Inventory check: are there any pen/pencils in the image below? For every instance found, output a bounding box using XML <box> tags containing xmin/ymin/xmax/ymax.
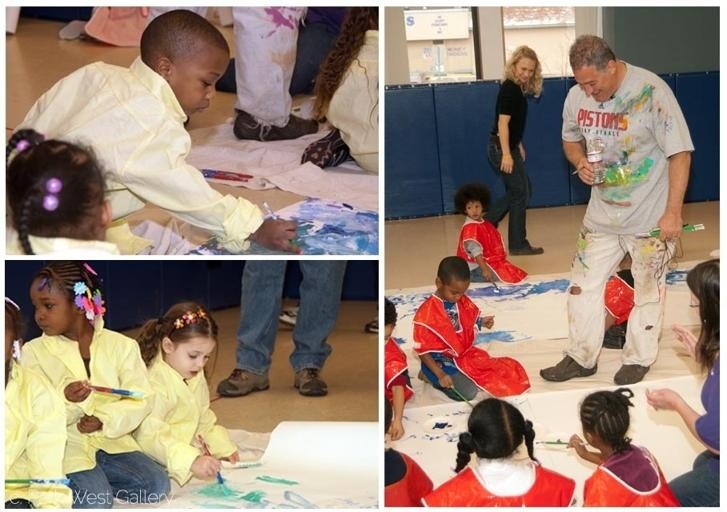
<box><xmin>262</xmin><ymin>201</ymin><xmax>296</xmax><ymax>245</ymax></box>
<box><xmin>569</xmin><ymin>166</ymin><xmax>584</xmax><ymax>176</ymax></box>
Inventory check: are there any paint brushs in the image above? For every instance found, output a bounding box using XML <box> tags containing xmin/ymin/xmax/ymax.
<box><xmin>493</xmin><ymin>282</ymin><xmax>499</xmax><ymax>291</ymax></box>
<box><xmin>535</xmin><ymin>441</ymin><xmax>591</xmax><ymax>445</ymax></box>
<box><xmin>449</xmin><ymin>385</ymin><xmax>475</xmax><ymax>410</ymax></box>
<box><xmin>89</xmin><ymin>386</ymin><xmax>144</xmax><ymax>397</ymax></box>
<box><xmin>5</xmin><ymin>479</ymin><xmax>75</xmax><ymax>488</ymax></box>
<box><xmin>199</xmin><ymin>434</ymin><xmax>231</xmax><ymax>495</ymax></box>
<box><xmin>634</xmin><ymin>223</ymin><xmax>705</xmax><ymax>238</ymax></box>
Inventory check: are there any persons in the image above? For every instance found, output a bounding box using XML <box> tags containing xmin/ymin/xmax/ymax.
<box><xmin>411</xmin><ymin>253</ymin><xmax>496</xmax><ymax>402</ymax></box>
<box><xmin>644</xmin><ymin>256</ymin><xmax>718</xmax><ymax>505</ymax></box>
<box><xmin>5</xmin><ymin>294</ymin><xmax>74</xmax><ymax>509</ymax></box>
<box><xmin>57</xmin><ymin>9</ymin><xmax>379</xmax><ymax>179</ymax></box>
<box><xmin>418</xmin><ymin>396</ymin><xmax>582</xmax><ymax>507</ymax></box>
<box><xmin>7</xmin><ymin>126</ymin><xmax>126</xmax><ymax>256</ymax></box>
<box><xmin>19</xmin><ymin>259</ymin><xmax>170</xmax><ymax>510</ymax></box>
<box><xmin>213</xmin><ymin>259</ymin><xmax>350</xmax><ymax>397</ymax></box>
<box><xmin>276</xmin><ymin>273</ymin><xmax>378</xmax><ymax>334</ymax></box>
<box><xmin>7</xmin><ymin>9</ymin><xmax>308</xmax><ymax>256</ymax></box>
<box><xmin>383</xmin><ymin>397</ymin><xmax>433</xmax><ymax>506</ymax></box>
<box><xmin>451</xmin><ymin>182</ymin><xmax>529</xmax><ymax>286</ymax></box>
<box><xmin>564</xmin><ymin>385</ymin><xmax>684</xmax><ymax>506</ymax></box>
<box><xmin>481</xmin><ymin>45</ymin><xmax>545</xmax><ymax>256</ymax></box>
<box><xmin>603</xmin><ymin>250</ymin><xmax>635</xmax><ymax>350</ymax></box>
<box><xmin>383</xmin><ymin>295</ymin><xmax>414</xmax><ymax>441</ymax></box>
<box><xmin>537</xmin><ymin>33</ymin><xmax>694</xmax><ymax>386</ymax></box>
<box><xmin>128</xmin><ymin>298</ymin><xmax>241</xmax><ymax>487</ymax></box>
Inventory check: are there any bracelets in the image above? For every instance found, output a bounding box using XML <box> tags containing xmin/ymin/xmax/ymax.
<box><xmin>502</xmin><ymin>155</ymin><xmax>512</xmax><ymax>160</ymax></box>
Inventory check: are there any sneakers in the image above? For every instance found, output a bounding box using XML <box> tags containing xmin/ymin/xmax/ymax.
<box><xmin>615</xmin><ymin>360</ymin><xmax>648</xmax><ymax>385</ymax></box>
<box><xmin>294</xmin><ymin>367</ymin><xmax>329</xmax><ymax>397</ymax></box>
<box><xmin>278</xmin><ymin>306</ymin><xmax>300</xmax><ymax>326</ymax></box>
<box><xmin>365</xmin><ymin>320</ymin><xmax>379</xmax><ymax>333</ymax></box>
<box><xmin>230</xmin><ymin>111</ymin><xmax>319</xmax><ymax>141</ymax></box>
<box><xmin>418</xmin><ymin>370</ymin><xmax>432</xmax><ymax>384</ymax></box>
<box><xmin>508</xmin><ymin>245</ymin><xmax>543</xmax><ymax>255</ymax></box>
<box><xmin>541</xmin><ymin>356</ymin><xmax>597</xmax><ymax>380</ymax></box>
<box><xmin>301</xmin><ymin>126</ymin><xmax>352</xmax><ymax>170</ymax></box>
<box><xmin>217</xmin><ymin>369</ymin><xmax>269</xmax><ymax>398</ymax></box>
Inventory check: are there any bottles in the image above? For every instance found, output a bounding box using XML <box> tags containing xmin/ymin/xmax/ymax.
<box><xmin>585</xmin><ymin>137</ymin><xmax>606</xmax><ymax>185</ymax></box>
